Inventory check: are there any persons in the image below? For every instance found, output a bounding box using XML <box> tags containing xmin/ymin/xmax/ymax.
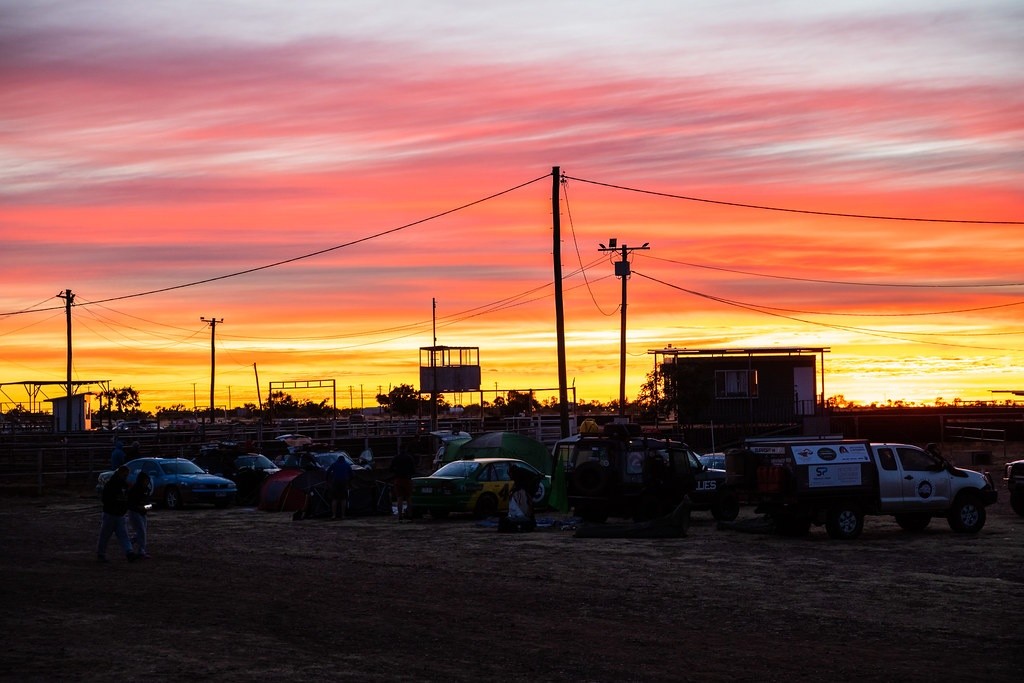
<box><xmin>207</xmin><ymin>439</ymin><xmax>354</xmax><ymax>521</ymax></box>
<box><xmin>431</xmin><ymin>441</ymin><xmax>447</xmax><ymax>471</ymax></box>
<box><xmin>926</xmin><ymin>443</ymin><xmax>945</xmax><ymax>459</ymax></box>
<box><xmin>390</xmin><ymin>444</ymin><xmax>415</xmax><ymax>524</ymax></box>
<box><xmin>95</xmin><ymin>442</ymin><xmax>153</xmax><ymax>561</ymax></box>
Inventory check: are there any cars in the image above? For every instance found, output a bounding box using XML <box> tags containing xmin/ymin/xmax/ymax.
<box><xmin>94</xmin><ymin>457</ymin><xmax>238</xmax><ymax>509</ymax></box>
<box><xmin>95</xmin><ymin>419</ymin><xmax>165</xmax><ymax>433</ymax></box>
<box><xmin>410</xmin><ymin>456</ymin><xmax>553</xmax><ymax>520</ymax></box>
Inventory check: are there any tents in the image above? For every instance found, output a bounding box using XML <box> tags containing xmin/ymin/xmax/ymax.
<box><xmin>455</xmin><ymin>431</ymin><xmax>567</xmax><ymax>512</ymax></box>
<box><xmin>260</xmin><ymin>467</ymin><xmax>390</xmax><ymax>518</ymax></box>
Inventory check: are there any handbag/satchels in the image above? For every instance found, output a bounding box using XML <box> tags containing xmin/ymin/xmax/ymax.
<box><xmin>499</xmin><ymin>515</ymin><xmax>531</xmax><ymax>532</ymax></box>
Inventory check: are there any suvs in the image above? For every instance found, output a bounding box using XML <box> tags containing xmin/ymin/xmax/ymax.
<box><xmin>566</xmin><ymin>419</ymin><xmax>741</xmax><ymax>525</ymax></box>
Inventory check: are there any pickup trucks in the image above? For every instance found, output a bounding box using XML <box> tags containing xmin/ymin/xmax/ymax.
<box><xmin>720</xmin><ymin>434</ymin><xmax>997</xmax><ymax>541</ymax></box>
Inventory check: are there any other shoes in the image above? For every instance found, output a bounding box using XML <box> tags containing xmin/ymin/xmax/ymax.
<box><xmin>128</xmin><ymin>552</ymin><xmax>144</xmax><ymax>561</ymax></box>
<box><xmin>144</xmin><ymin>554</ymin><xmax>152</xmax><ymax>559</ymax></box>
<box><xmin>96</xmin><ymin>555</ymin><xmax>108</xmax><ymax>562</ymax></box>
